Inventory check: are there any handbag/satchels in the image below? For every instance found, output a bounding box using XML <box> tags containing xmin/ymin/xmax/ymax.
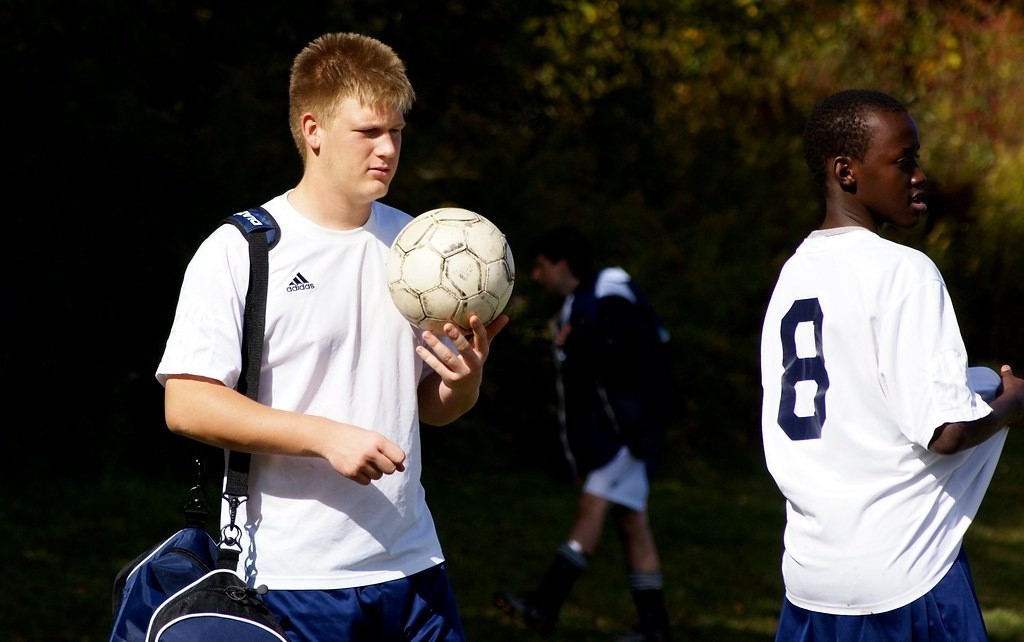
<box><xmin>106</xmin><ymin>524</ymin><xmax>291</xmax><ymax>642</ymax></box>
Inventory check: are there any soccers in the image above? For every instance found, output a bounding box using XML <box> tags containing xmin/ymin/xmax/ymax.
<box><xmin>383</xmin><ymin>205</ymin><xmax>520</xmax><ymax>339</ymax></box>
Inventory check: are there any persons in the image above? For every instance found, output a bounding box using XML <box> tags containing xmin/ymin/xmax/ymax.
<box><xmin>491</xmin><ymin>240</ymin><xmax>683</xmax><ymax>642</ymax></box>
<box><xmin>760</xmin><ymin>89</ymin><xmax>1024</xmax><ymax>642</ymax></box>
<box><xmin>156</xmin><ymin>32</ymin><xmax>509</xmax><ymax>642</ymax></box>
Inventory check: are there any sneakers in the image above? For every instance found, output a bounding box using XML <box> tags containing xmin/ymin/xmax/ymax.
<box><xmin>491</xmin><ymin>590</ymin><xmax>562</xmax><ymax>639</ymax></box>
<box><xmin>616</xmin><ymin>633</ymin><xmax>674</xmax><ymax>642</ymax></box>
